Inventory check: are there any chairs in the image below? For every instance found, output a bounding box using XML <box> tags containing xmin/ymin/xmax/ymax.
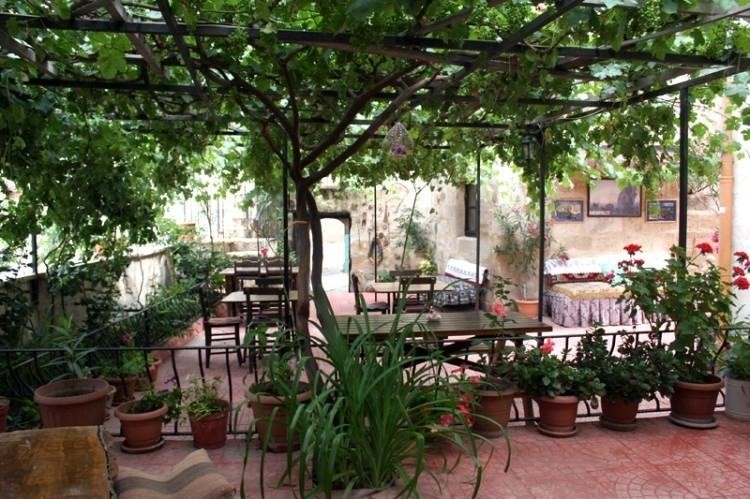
<box><xmin>201</xmin><ymin>256</ymin><xmax>437</xmax><ymax>371</ymax></box>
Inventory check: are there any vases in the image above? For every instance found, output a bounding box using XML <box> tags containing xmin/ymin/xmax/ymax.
<box><xmin>534</xmin><ymin>394</ymin><xmax>581</xmax><ymax>437</ymax></box>
<box><xmin>668</xmin><ymin>374</ymin><xmax>726</xmax><ymax>429</ymax></box>
<box><xmin>463</xmin><ymin>377</ymin><xmax>517</xmax><ymax>436</ymax></box>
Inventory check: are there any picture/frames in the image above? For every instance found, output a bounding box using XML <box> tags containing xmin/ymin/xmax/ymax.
<box><xmin>586</xmin><ymin>176</ymin><xmax>641</xmax><ymax>218</ymax></box>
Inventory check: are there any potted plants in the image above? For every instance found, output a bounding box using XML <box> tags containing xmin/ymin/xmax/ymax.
<box><xmin>35</xmin><ymin>318</ymin><xmax>312</xmax><ymax>453</ymax></box>
<box><xmin>586</xmin><ymin>326</ymin><xmax>660</xmax><ymax>425</ymax></box>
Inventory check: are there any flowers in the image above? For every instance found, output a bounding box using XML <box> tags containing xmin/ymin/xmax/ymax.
<box><xmin>527</xmin><ymin>340</ymin><xmax>587</xmax><ymax>398</ymax></box>
<box><xmin>613</xmin><ymin>242</ymin><xmax>750</xmax><ymax>377</ymax></box>
<box><xmin>437</xmin><ymin>301</ymin><xmax>522</xmax><ymax>427</ymax></box>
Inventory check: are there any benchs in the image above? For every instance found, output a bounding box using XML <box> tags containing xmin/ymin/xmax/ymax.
<box><xmin>434</xmin><ymin>257</ymin><xmax>488</xmax><ymax>306</ymax></box>
